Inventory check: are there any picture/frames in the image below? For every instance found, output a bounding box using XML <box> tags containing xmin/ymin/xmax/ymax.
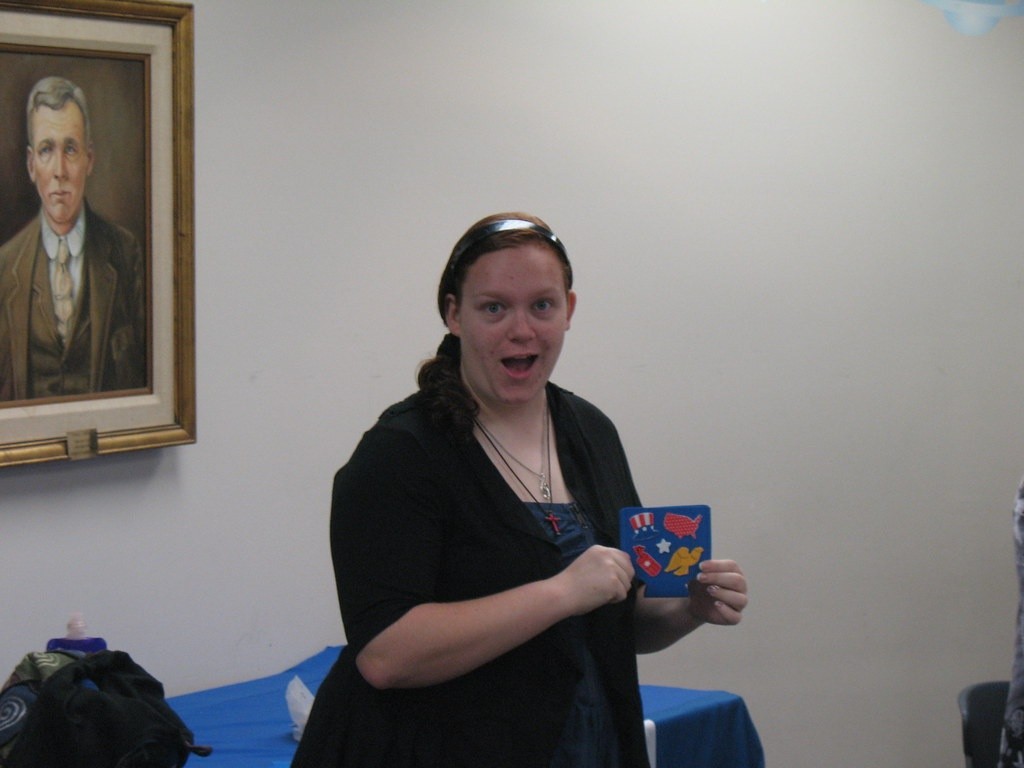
<box><xmin>0</xmin><ymin>0</ymin><xmax>197</xmax><ymax>466</ymax></box>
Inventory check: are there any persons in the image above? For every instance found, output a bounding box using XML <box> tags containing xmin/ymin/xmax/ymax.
<box><xmin>1005</xmin><ymin>479</ymin><xmax>1024</xmax><ymax>750</ymax></box>
<box><xmin>288</xmin><ymin>214</ymin><xmax>748</xmax><ymax>768</ymax></box>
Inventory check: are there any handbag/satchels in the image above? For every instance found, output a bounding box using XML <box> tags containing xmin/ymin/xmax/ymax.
<box><xmin>8</xmin><ymin>651</ymin><xmax>212</xmax><ymax>768</ymax></box>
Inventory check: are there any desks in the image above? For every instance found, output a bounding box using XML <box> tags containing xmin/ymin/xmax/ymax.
<box><xmin>164</xmin><ymin>644</ymin><xmax>765</xmax><ymax>768</ymax></box>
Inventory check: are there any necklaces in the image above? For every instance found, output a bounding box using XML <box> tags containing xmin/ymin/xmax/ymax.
<box><xmin>474</xmin><ymin>384</ymin><xmax>561</xmax><ymax>537</ymax></box>
